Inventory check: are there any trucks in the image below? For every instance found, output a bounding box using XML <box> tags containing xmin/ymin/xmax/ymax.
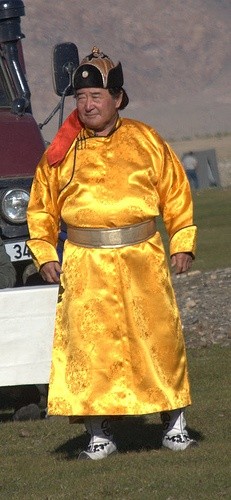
<box><xmin>0</xmin><ymin>0</ymin><xmax>79</xmax><ymax>412</ymax></box>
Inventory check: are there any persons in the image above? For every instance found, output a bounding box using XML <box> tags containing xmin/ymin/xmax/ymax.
<box><xmin>25</xmin><ymin>46</ymin><xmax>198</xmax><ymax>460</ymax></box>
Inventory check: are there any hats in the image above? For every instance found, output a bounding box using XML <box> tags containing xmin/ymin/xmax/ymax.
<box><xmin>71</xmin><ymin>46</ymin><xmax>123</xmax><ymax>90</ymax></box>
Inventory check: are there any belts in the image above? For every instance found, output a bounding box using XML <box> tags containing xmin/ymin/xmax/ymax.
<box><xmin>66</xmin><ymin>219</ymin><xmax>155</xmax><ymax>249</ymax></box>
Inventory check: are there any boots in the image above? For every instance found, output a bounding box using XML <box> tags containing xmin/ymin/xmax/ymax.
<box><xmin>160</xmin><ymin>406</ymin><xmax>200</xmax><ymax>451</ymax></box>
<box><xmin>77</xmin><ymin>413</ymin><xmax>117</xmax><ymax>460</ymax></box>
